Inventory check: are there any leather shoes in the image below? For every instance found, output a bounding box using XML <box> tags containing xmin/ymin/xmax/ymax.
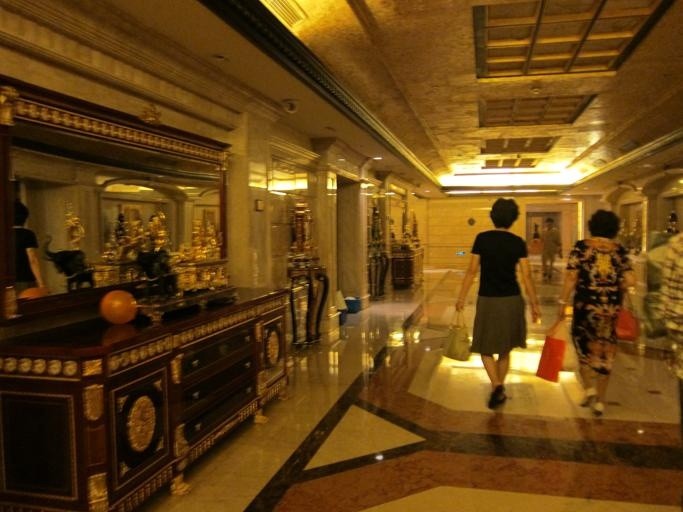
<box><xmin>489</xmin><ymin>383</ymin><xmax>508</xmax><ymax>410</ymax></box>
<box><xmin>578</xmin><ymin>385</ymin><xmax>608</xmax><ymax>418</ymax></box>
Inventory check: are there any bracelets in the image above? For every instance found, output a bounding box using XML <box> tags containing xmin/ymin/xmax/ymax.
<box><xmin>557</xmin><ymin>299</ymin><xmax>567</xmax><ymax>305</ymax></box>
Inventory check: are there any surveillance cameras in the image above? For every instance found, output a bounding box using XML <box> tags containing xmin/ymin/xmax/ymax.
<box><xmin>282</xmin><ymin>99</ymin><xmax>300</xmax><ymax>114</ymax></box>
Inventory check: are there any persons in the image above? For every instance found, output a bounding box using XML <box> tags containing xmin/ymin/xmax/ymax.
<box><xmin>534</xmin><ymin>218</ymin><xmax>563</xmax><ymax>278</ymax></box>
<box><xmin>556</xmin><ymin>210</ymin><xmax>637</xmax><ymax>415</ymax></box>
<box><xmin>13</xmin><ymin>201</ymin><xmax>46</xmax><ymax>288</ymax></box>
<box><xmin>454</xmin><ymin>196</ymin><xmax>540</xmax><ymax>411</ymax></box>
<box><xmin>654</xmin><ymin>227</ymin><xmax>683</xmax><ymax>447</ymax></box>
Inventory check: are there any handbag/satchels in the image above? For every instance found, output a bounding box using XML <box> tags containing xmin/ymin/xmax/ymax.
<box><xmin>615</xmin><ymin>289</ymin><xmax>642</xmax><ymax>342</ymax></box>
<box><xmin>442</xmin><ymin>309</ymin><xmax>472</xmax><ymax>361</ymax></box>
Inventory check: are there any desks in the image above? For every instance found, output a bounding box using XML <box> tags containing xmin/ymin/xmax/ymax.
<box><xmin>285</xmin><ymin>264</ymin><xmax>330</xmax><ymax>346</ymax></box>
<box><xmin>367</xmin><ymin>250</ymin><xmax>389</xmax><ymax>301</ymax></box>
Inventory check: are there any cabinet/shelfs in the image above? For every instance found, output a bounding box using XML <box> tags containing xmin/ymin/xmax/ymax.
<box><xmin>0</xmin><ymin>287</ymin><xmax>294</xmax><ymax>511</ymax></box>
<box><xmin>392</xmin><ymin>247</ymin><xmax>426</xmax><ymax>289</ymax></box>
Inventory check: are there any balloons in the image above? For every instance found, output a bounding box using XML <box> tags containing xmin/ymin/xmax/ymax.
<box><xmin>18</xmin><ymin>286</ymin><xmax>45</xmax><ymax>299</ymax></box>
<box><xmin>98</xmin><ymin>290</ymin><xmax>139</xmax><ymax>325</ymax></box>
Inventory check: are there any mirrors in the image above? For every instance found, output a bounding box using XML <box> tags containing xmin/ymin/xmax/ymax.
<box><xmin>0</xmin><ymin>73</ymin><xmax>231</xmax><ymax>324</ymax></box>
